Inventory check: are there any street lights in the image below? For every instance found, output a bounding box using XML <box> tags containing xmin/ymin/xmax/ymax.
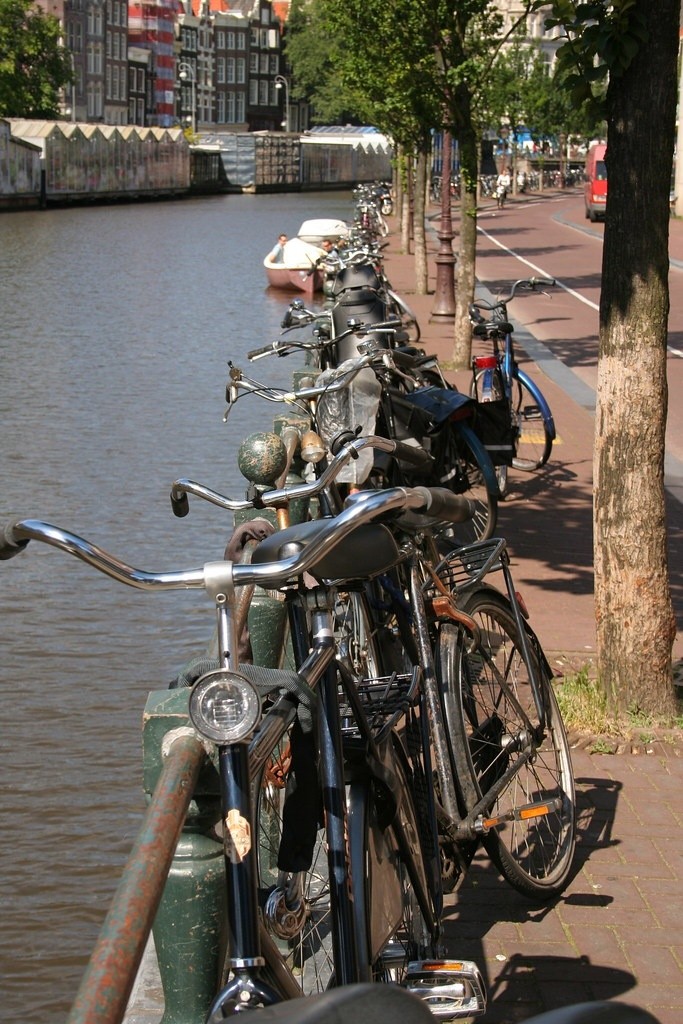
<box><xmin>274</xmin><ymin>75</ymin><xmax>291</xmax><ymax>133</ymax></box>
<box><xmin>178</xmin><ymin>62</ymin><xmax>199</xmax><ymax>136</ymax></box>
<box><xmin>52</xmin><ymin>44</ymin><xmax>77</xmax><ymax>126</ymax></box>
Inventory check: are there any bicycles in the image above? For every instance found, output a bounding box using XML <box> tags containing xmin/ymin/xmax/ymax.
<box><xmin>0</xmin><ymin>164</ymin><xmax>588</xmax><ymax>1024</ymax></box>
<box><xmin>493</xmin><ymin>181</ymin><xmax>507</xmax><ymax>214</ymax></box>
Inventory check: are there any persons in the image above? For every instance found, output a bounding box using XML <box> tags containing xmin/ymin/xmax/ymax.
<box><xmin>496</xmin><ymin>170</ymin><xmax>510</xmax><ymax>210</ymax></box>
<box><xmin>270</xmin><ymin>234</ymin><xmax>287</xmax><ymax>263</ymax></box>
<box><xmin>322</xmin><ymin>239</ymin><xmax>348</xmax><ymax>272</ymax></box>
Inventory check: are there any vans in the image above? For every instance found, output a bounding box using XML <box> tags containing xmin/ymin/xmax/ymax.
<box><xmin>581</xmin><ymin>143</ymin><xmax>609</xmax><ymax>222</ymax></box>
